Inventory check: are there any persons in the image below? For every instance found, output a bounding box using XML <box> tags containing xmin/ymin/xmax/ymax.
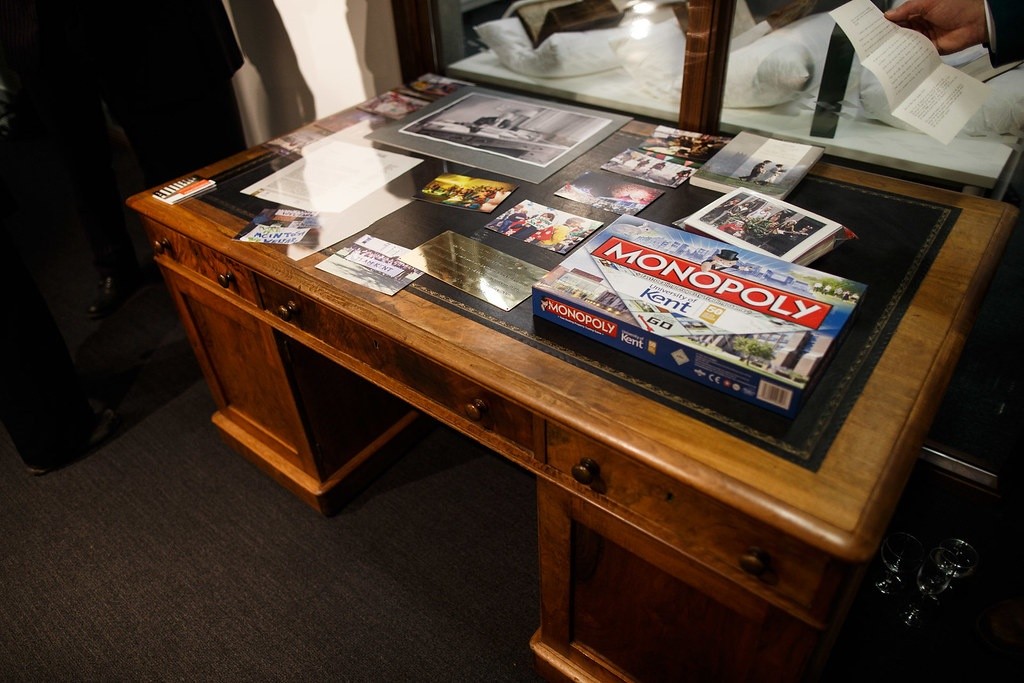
<box><xmin>608</xmin><ymin>151</ymin><xmax>692</xmax><ymax>186</ymax></box>
<box><xmin>701</xmin><ymin>198</ymin><xmax>813</xmax><ymax>242</ymax></box>
<box><xmin>884</xmin><ymin>0</ymin><xmax>1024</xmax><ymax>69</ymax></box>
<box><xmin>342</xmin><ymin>245</ymin><xmax>415</xmax><ymax>282</ymax></box>
<box><xmin>424</xmin><ymin>179</ymin><xmax>497</xmax><ymax>211</ymax></box>
<box><xmin>740</xmin><ymin>159</ymin><xmax>788</xmax><ymax>186</ymax></box>
<box><xmin>642</xmin><ymin>132</ymin><xmax>730</xmax><ymax>160</ymax></box>
<box><xmin>470</xmin><ymin>112</ymin><xmax>514</xmax><ymax>133</ymax></box>
<box><xmin>489</xmin><ymin>204</ymin><xmax>596</xmax><ymax>252</ymax></box>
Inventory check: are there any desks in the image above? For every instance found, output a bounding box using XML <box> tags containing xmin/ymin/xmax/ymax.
<box><xmin>391</xmin><ymin>0</ymin><xmax>1024</xmax><ymax>202</ymax></box>
<box><xmin>125</xmin><ymin>70</ymin><xmax>1021</xmax><ymax>683</ymax></box>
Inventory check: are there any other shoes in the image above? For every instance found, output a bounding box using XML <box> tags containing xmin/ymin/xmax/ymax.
<box><xmin>27</xmin><ymin>408</ymin><xmax>119</xmax><ymax>475</ymax></box>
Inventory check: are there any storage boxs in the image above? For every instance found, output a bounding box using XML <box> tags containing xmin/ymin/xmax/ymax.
<box><xmin>532</xmin><ymin>212</ymin><xmax>869</xmax><ymax>420</ymax></box>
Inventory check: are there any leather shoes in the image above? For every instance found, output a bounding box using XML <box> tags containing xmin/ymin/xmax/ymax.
<box><xmin>88</xmin><ymin>275</ymin><xmax>132</xmax><ymax>317</ymax></box>
